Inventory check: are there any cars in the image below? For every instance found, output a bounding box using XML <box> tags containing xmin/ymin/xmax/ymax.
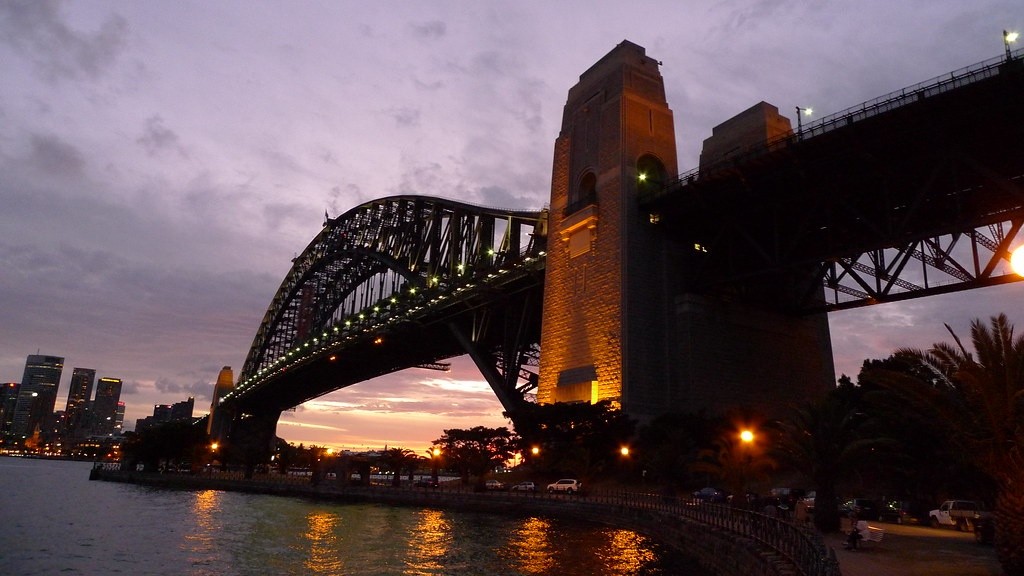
<box><xmin>512</xmin><ymin>481</ymin><xmax>535</xmax><ymax>492</ymax></box>
<box><xmin>751</xmin><ymin>493</ymin><xmax>798</xmax><ymax>513</ymax></box>
<box><xmin>801</xmin><ymin>491</ymin><xmax>817</xmax><ymax>513</ymax></box>
<box><xmin>876</xmin><ymin>505</ymin><xmax>924</xmax><ymax>525</ymax></box>
<box><xmin>485</xmin><ymin>479</ymin><xmax>504</xmax><ymax>491</ymax></box>
<box><xmin>929</xmin><ymin>499</ymin><xmax>984</xmax><ymax>533</ymax></box>
<box><xmin>693</xmin><ymin>486</ymin><xmax>726</xmax><ymax>503</ymax></box>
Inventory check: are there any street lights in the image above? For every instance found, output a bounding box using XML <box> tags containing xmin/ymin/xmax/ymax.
<box><xmin>1002</xmin><ymin>29</ymin><xmax>1017</xmax><ymax>62</ymax></box>
<box><xmin>796</xmin><ymin>105</ymin><xmax>812</xmax><ymax>143</ymax></box>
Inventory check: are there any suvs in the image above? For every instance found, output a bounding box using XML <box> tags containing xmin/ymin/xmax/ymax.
<box><xmin>547</xmin><ymin>479</ymin><xmax>583</xmax><ymax>495</ymax></box>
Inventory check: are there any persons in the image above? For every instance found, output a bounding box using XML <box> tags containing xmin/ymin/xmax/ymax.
<box><xmin>847</xmin><ymin>503</ymin><xmax>859</xmax><ymax>529</ymax></box>
<box><xmin>792</xmin><ymin>496</ymin><xmax>809</xmax><ymax>527</ymax></box>
<box><xmin>744</xmin><ymin>487</ymin><xmax>757</xmax><ymax>511</ymax></box>
<box><xmin>576</xmin><ymin>480</ymin><xmax>583</xmax><ymax>496</ymax></box>
<box><xmin>763</xmin><ymin>491</ymin><xmax>779</xmax><ymax>517</ymax></box>
